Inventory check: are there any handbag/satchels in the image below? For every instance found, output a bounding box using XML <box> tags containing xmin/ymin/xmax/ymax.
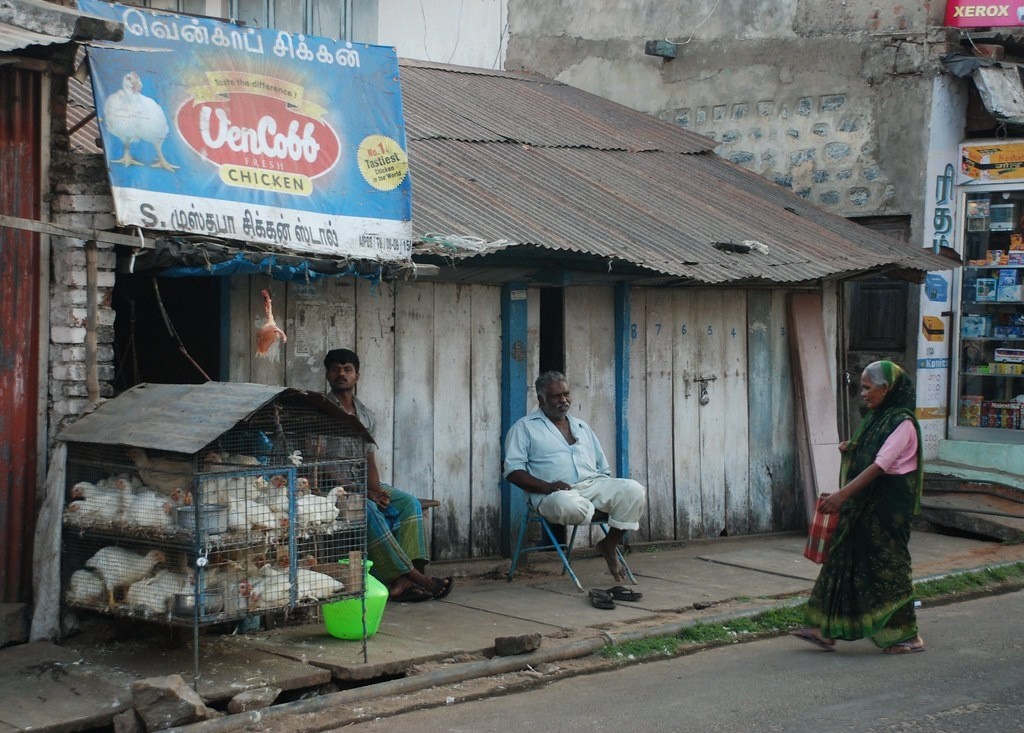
<box><xmin>804</xmin><ymin>492</ymin><xmax>839</xmax><ymax>564</ymax></box>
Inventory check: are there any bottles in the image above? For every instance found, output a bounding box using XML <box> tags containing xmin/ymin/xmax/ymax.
<box><xmin>321</xmin><ymin>559</ymin><xmax>389</xmax><ymax>640</ymax></box>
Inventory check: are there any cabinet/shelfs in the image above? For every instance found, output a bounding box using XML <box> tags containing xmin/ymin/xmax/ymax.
<box><xmin>948</xmin><ymin>139</ymin><xmax>1024</xmax><ymax>445</ymax></box>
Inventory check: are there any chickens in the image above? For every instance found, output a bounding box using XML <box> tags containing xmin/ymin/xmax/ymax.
<box><xmin>58</xmin><ymin>448</ymin><xmax>351</xmax><ymax>618</ymax></box>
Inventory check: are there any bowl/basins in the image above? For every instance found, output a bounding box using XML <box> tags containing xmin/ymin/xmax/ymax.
<box><xmin>175</xmin><ymin>503</ymin><xmax>228</xmax><ymax>534</ymax></box>
<box><xmin>174</xmin><ymin>592</ymin><xmax>224</xmax><ymax>618</ymax></box>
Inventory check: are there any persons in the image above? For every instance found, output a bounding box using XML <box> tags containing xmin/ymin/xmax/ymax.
<box><xmin>502</xmin><ymin>369</ymin><xmax>647</xmax><ymax>582</ymax></box>
<box><xmin>785</xmin><ymin>358</ymin><xmax>927</xmax><ymax>655</ymax></box>
<box><xmin>309</xmin><ymin>348</ymin><xmax>455</xmax><ymax>603</ymax></box>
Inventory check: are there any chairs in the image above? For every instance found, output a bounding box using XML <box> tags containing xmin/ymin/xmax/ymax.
<box><xmin>508</xmin><ymin>486</ymin><xmax>638</xmax><ymax>595</ymax></box>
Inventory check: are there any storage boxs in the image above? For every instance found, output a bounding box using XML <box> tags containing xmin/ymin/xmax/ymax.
<box><xmin>998</xmin><ymin>269</ymin><xmax>1017</xmax><ymax>286</ymax></box>
<box><xmin>997</xmin><ymin>286</ymin><xmax>1023</xmax><ymax>302</ymax></box>
<box><xmin>966</xmin><ymin>199</ymin><xmax>990</xmax><ymax>217</ymax></box>
<box><xmin>981</xmin><ymin>401</ymin><xmax>1024</xmax><ymax>430</ymax></box>
<box><xmin>960</xmin><ymin>317</ymin><xmax>991</xmax><ymax>337</ymax></box>
<box><xmin>976</xmin><ymin>278</ymin><xmax>997</xmax><ymax>302</ymax></box>
<box><xmin>958</xmin><ymin>396</ymin><xmax>984</xmax><ymax>427</ymax></box>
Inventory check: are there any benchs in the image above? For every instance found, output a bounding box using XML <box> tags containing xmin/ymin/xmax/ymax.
<box><xmin>418</xmin><ymin>496</ymin><xmax>442</xmax><ymax>570</ymax></box>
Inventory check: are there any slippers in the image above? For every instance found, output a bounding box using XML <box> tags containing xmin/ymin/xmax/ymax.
<box><xmin>431</xmin><ymin>576</ymin><xmax>455</xmax><ymax>598</ymax></box>
<box><xmin>792</xmin><ymin>628</ymin><xmax>836</xmax><ymax>651</ymax></box>
<box><xmin>883</xmin><ymin>642</ymin><xmax>926</xmax><ymax>654</ymax></box>
<box><xmin>390</xmin><ymin>584</ymin><xmax>433</xmax><ymax>603</ymax></box>
<box><xmin>588</xmin><ymin>589</ymin><xmax>616</xmax><ymax>608</ymax></box>
<box><xmin>607</xmin><ymin>585</ymin><xmax>642</xmax><ymax>600</ymax></box>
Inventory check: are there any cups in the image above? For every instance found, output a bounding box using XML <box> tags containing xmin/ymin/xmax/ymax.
<box><xmin>962</xmin><ymin>148</ymin><xmax>971</xmax><ymax>174</ymax></box>
<box><xmin>981</xmin><ymin>154</ymin><xmax>991</xmax><ymax>179</ymax></box>
